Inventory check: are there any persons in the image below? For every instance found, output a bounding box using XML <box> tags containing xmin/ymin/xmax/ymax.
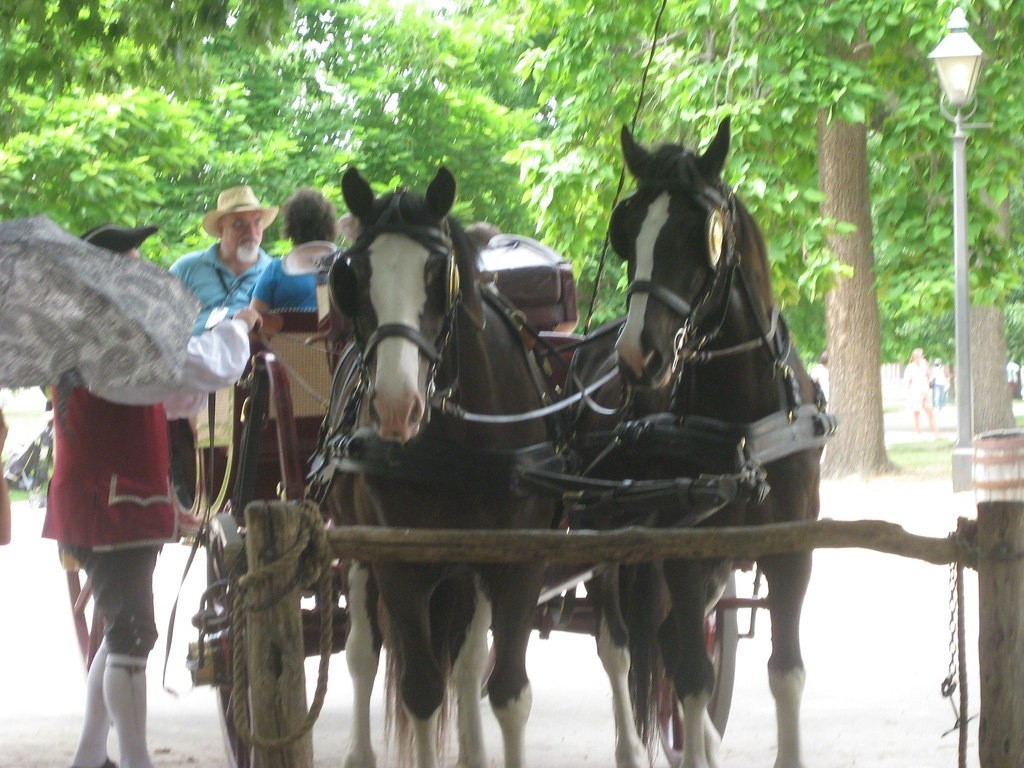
<box><xmin>251</xmin><ymin>185</ymin><xmax>344</xmax><ymax>306</ymax></box>
<box><xmin>931</xmin><ymin>355</ymin><xmax>1024</xmax><ymax>406</ymax></box>
<box><xmin>36</xmin><ymin>220</ymin><xmax>262</xmax><ymax>768</ymax></box>
<box><xmin>164</xmin><ymin>185</ymin><xmax>281</xmax><ymax>508</ymax></box>
<box><xmin>809</xmin><ymin>351</ymin><xmax>830</xmax><ymax>408</ymax></box>
<box><xmin>464</xmin><ymin>221</ymin><xmax>502</xmax><ymax>248</ymax></box>
<box><xmin>903</xmin><ymin>348</ymin><xmax>938</xmax><ymax>431</ymax></box>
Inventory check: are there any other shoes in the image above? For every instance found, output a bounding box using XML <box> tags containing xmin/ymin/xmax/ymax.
<box><xmin>103</xmin><ymin>758</ymin><xmax>120</xmax><ymax>768</ymax></box>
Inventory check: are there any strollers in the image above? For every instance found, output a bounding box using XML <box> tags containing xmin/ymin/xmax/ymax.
<box><xmin>3</xmin><ymin>418</ymin><xmax>54</xmax><ymax>509</ymax></box>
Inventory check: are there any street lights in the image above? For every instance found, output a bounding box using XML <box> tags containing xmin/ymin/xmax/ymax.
<box><xmin>928</xmin><ymin>5</ymin><xmax>985</xmax><ymax>518</ymax></box>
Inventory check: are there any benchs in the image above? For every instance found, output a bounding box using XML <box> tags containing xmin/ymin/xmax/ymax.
<box><xmin>215</xmin><ymin>265</ymin><xmax>580</xmax><ymax>518</ymax></box>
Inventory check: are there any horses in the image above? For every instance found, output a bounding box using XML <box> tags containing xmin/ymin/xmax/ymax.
<box><xmin>322</xmin><ymin>162</ymin><xmax>556</xmax><ymax>768</ymax></box>
<box><xmin>562</xmin><ymin>112</ymin><xmax>828</xmax><ymax>768</ymax></box>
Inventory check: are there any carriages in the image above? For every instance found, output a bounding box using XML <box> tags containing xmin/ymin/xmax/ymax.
<box><xmin>174</xmin><ymin>113</ymin><xmax>838</xmax><ymax>768</ymax></box>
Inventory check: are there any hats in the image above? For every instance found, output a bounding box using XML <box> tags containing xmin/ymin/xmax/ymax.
<box><xmin>81</xmin><ymin>226</ymin><xmax>157</xmax><ymax>252</ymax></box>
<box><xmin>202</xmin><ymin>185</ymin><xmax>280</xmax><ymax>239</ymax></box>
